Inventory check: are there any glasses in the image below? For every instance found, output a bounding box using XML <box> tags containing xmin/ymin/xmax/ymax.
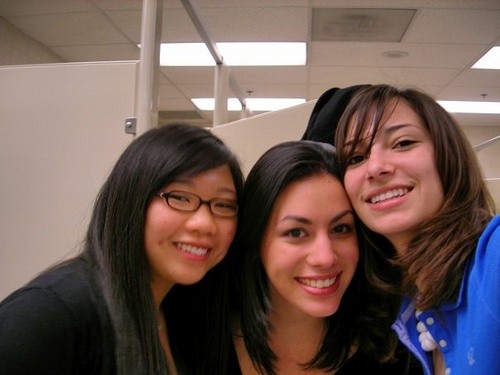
<box><xmin>156</xmin><ymin>190</ymin><xmax>239</xmax><ymax>218</ymax></box>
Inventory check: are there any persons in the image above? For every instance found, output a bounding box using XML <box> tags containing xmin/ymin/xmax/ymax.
<box><xmin>227</xmin><ymin>140</ymin><xmax>370</xmax><ymax>375</ymax></box>
<box><xmin>336</xmin><ymin>84</ymin><xmax>500</xmax><ymax>375</ymax></box>
<box><xmin>0</xmin><ymin>124</ymin><xmax>245</xmax><ymax>375</ymax></box>
